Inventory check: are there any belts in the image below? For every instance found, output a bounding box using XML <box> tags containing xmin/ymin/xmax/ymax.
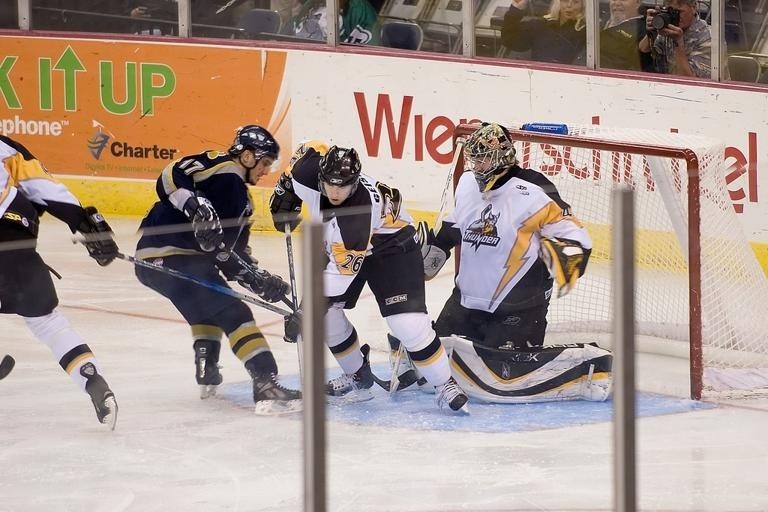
<box><xmin>249</xmin><ymin>268</ymin><xmax>293</xmax><ymax>303</ymax></box>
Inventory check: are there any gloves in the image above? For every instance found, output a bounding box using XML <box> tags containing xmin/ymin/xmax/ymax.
<box><xmin>68</xmin><ymin>202</ymin><xmax>119</xmax><ymax>267</ymax></box>
<box><xmin>180</xmin><ymin>194</ymin><xmax>224</xmax><ymax>269</ymax></box>
<box><xmin>271</xmin><ymin>171</ymin><xmax>306</xmax><ymax>232</ymax></box>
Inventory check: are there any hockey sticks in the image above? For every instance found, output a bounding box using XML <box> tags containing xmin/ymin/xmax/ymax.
<box><xmin>387</xmin><ymin>136</ymin><xmax>466</xmax><ymax>398</ymax></box>
<box><xmin>220</xmin><ymin>242</ymin><xmax>418</xmax><ymax>392</ymax></box>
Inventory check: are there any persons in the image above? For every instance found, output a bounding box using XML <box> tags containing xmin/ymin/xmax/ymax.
<box><xmin>0</xmin><ymin>134</ymin><xmax>119</xmax><ymax>433</ymax></box>
<box><xmin>420</xmin><ymin>120</ymin><xmax>614</xmax><ymax>403</ymax></box>
<box><xmin>135</xmin><ymin>124</ymin><xmax>303</xmax><ymax>416</ymax></box>
<box><xmin>66</xmin><ymin>0</ymin><xmax>732</xmax><ymax>81</ymax></box>
<box><xmin>268</xmin><ymin>138</ymin><xmax>470</xmax><ymax>416</ymax></box>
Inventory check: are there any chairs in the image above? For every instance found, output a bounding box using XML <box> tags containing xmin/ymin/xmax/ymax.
<box><xmin>17</xmin><ymin>1</ymin><xmax>767</xmax><ymax>89</ymax></box>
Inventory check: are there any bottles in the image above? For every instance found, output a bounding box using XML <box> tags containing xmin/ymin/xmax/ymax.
<box><xmin>518</xmin><ymin>122</ymin><xmax>568</xmax><ymax>136</ymax></box>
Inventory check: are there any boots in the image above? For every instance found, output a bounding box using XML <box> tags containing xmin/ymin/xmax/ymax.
<box><xmin>325</xmin><ymin>362</ymin><xmax>374</xmax><ymax>397</ymax></box>
<box><xmin>433</xmin><ymin>376</ymin><xmax>468</xmax><ymax>414</ymax></box>
<box><xmin>249</xmin><ymin>373</ymin><xmax>312</xmax><ymax>402</ymax></box>
<box><xmin>84</xmin><ymin>367</ymin><xmax>116</xmax><ymax>425</ymax></box>
<box><xmin>189</xmin><ymin>339</ymin><xmax>223</xmax><ymax>386</ymax></box>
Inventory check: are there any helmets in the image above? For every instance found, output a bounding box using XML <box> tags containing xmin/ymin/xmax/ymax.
<box><xmin>226</xmin><ymin>121</ymin><xmax>274</xmax><ymax>179</ymax></box>
<box><xmin>319</xmin><ymin>145</ymin><xmax>359</xmax><ymax>202</ymax></box>
<box><xmin>464</xmin><ymin>119</ymin><xmax>518</xmax><ymax>179</ymax></box>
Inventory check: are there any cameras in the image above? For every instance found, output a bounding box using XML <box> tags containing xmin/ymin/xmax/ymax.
<box><xmin>651</xmin><ymin>6</ymin><xmax>680</xmax><ymax>30</ymax></box>
